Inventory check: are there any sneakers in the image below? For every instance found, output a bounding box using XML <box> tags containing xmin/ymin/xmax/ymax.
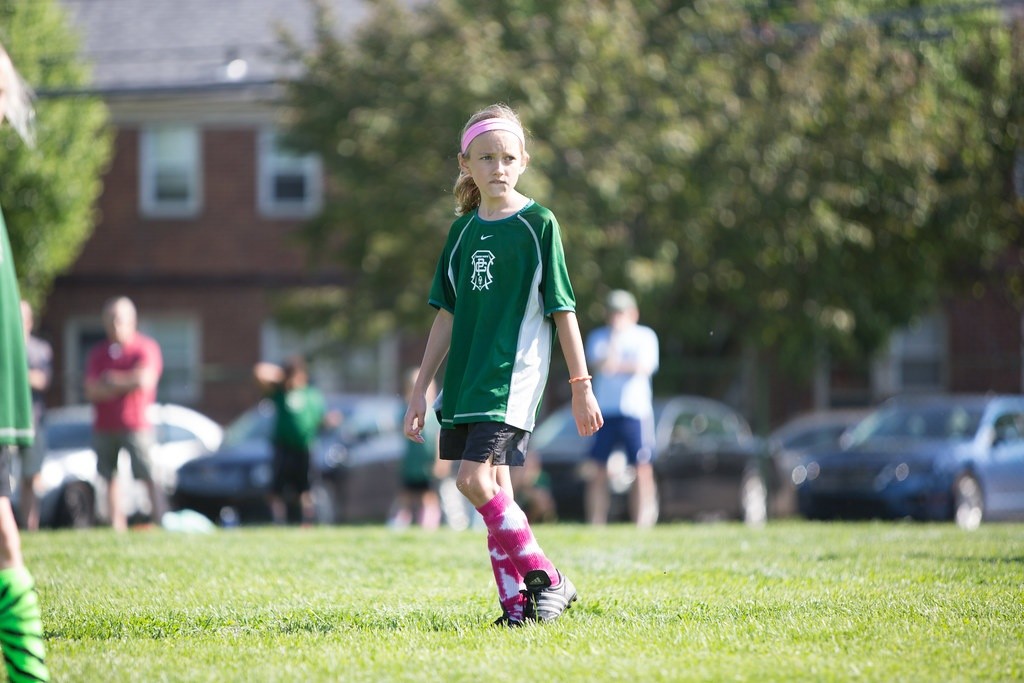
<box><xmin>490</xmin><ymin>597</ymin><xmax>525</xmax><ymax>628</ymax></box>
<box><xmin>521</xmin><ymin>565</ymin><xmax>578</xmax><ymax>624</ymax></box>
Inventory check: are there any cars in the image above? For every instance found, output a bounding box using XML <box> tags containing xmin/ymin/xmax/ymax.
<box><xmin>171</xmin><ymin>394</ymin><xmax>466</xmax><ymax>529</ymax></box>
<box><xmin>524</xmin><ymin>395</ymin><xmax>767</xmax><ymax>526</ymax></box>
<box><xmin>6</xmin><ymin>405</ymin><xmax>222</xmax><ymax>530</ymax></box>
<box><xmin>762</xmin><ymin>411</ymin><xmax>874</xmax><ymax>518</ymax></box>
<box><xmin>795</xmin><ymin>396</ymin><xmax>1023</xmax><ymax>530</ymax></box>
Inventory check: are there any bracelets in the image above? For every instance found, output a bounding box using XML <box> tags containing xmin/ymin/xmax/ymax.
<box><xmin>568</xmin><ymin>375</ymin><xmax>593</xmax><ymax>383</ymax></box>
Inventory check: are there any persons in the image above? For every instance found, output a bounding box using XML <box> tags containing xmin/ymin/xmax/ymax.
<box><xmin>584</xmin><ymin>289</ymin><xmax>660</xmax><ymax>526</ymax></box>
<box><xmin>404</xmin><ymin>101</ymin><xmax>604</xmax><ymax>628</ymax></box>
<box><xmin>254</xmin><ymin>358</ymin><xmax>341</xmax><ymax>527</ymax></box>
<box><xmin>83</xmin><ymin>295</ymin><xmax>167</xmax><ymax>530</ymax></box>
<box><xmin>395</xmin><ymin>368</ymin><xmax>454</xmax><ymax>529</ymax></box>
<box><xmin>9</xmin><ymin>300</ymin><xmax>53</xmax><ymax>531</ymax></box>
<box><xmin>0</xmin><ymin>201</ymin><xmax>51</xmax><ymax>683</ymax></box>
